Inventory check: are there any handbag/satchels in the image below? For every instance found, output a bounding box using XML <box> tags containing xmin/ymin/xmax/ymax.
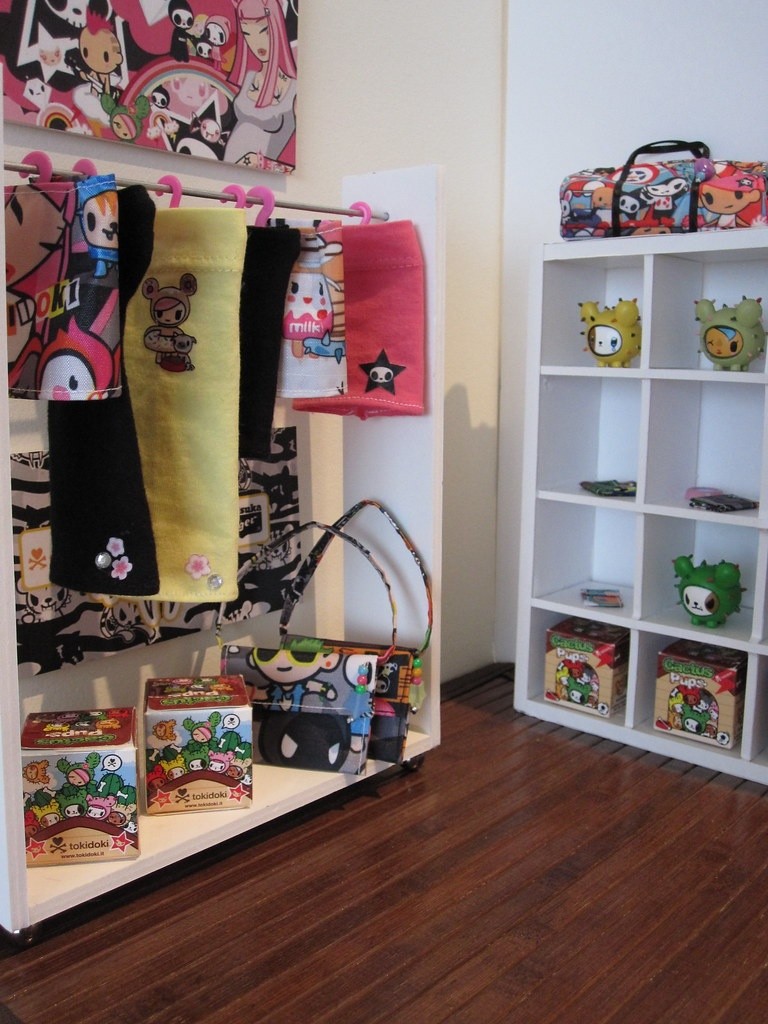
<box><xmin>216</xmin><ymin>499</ymin><xmax>433</xmax><ymax>775</ymax></box>
<box><xmin>560</xmin><ymin>141</ymin><xmax>768</xmax><ymax>241</ymax></box>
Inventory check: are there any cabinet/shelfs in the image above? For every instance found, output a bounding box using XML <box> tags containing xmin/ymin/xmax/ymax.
<box><xmin>513</xmin><ymin>228</ymin><xmax>768</xmax><ymax>786</ymax></box>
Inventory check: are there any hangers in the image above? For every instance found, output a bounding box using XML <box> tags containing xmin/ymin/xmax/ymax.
<box><xmin>71</xmin><ymin>158</ymin><xmax>97</xmax><ymax>177</ymax></box>
<box><xmin>221</xmin><ymin>184</ymin><xmax>247</xmax><ymax>209</ymax></box>
<box><xmin>349</xmin><ymin>201</ymin><xmax>371</xmax><ymax>226</ymax></box>
<box><xmin>156</xmin><ymin>175</ymin><xmax>182</xmax><ymax>208</ymax></box>
<box><xmin>247</xmin><ymin>186</ymin><xmax>275</xmax><ymax>227</ymax></box>
<box><xmin>18</xmin><ymin>152</ymin><xmax>52</xmax><ymax>183</ymax></box>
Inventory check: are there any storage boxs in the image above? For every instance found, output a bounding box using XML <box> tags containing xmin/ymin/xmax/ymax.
<box><xmin>544</xmin><ymin>616</ymin><xmax>630</xmax><ymax>717</ymax></box>
<box><xmin>143</xmin><ymin>675</ymin><xmax>254</xmax><ymax>815</ymax></box>
<box><xmin>653</xmin><ymin>639</ymin><xmax>747</xmax><ymax>750</ymax></box>
<box><xmin>21</xmin><ymin>706</ymin><xmax>141</xmax><ymax>868</ymax></box>
